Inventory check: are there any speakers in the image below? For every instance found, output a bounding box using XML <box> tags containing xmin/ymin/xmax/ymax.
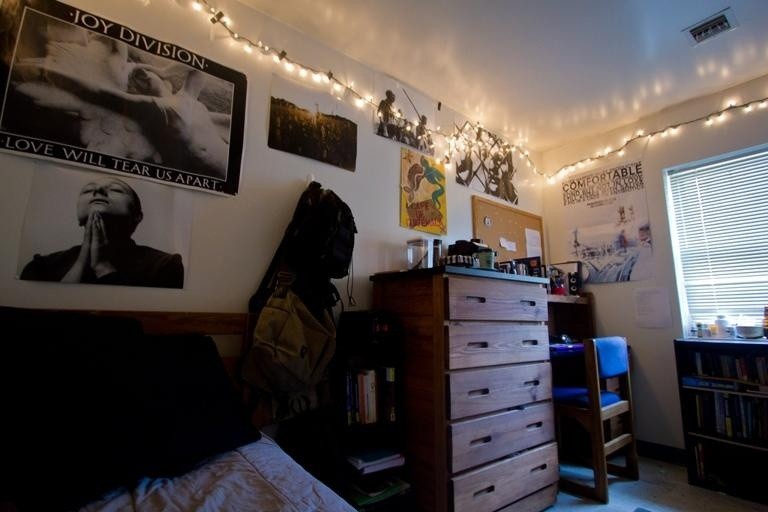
<box><xmin>568</xmin><ymin>271</ymin><xmax>581</xmax><ymax>296</ymax></box>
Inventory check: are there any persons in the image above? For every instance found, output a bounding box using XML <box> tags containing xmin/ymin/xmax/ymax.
<box><xmin>19</xmin><ymin>175</ymin><xmax>184</xmax><ymax>289</ymax></box>
<box><xmin>376</xmin><ymin>90</ymin><xmax>434</xmax><ymax>156</ymax></box>
<box><xmin>11</xmin><ymin>61</ymin><xmax>229</xmax><ymax>180</ymax></box>
<box><xmin>618</xmin><ymin>229</ymin><xmax>629</xmax><ymax>254</ymax></box>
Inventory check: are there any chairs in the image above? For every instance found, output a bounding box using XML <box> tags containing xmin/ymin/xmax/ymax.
<box><xmin>548</xmin><ymin>334</ymin><xmax>640</xmax><ymax>505</ymax></box>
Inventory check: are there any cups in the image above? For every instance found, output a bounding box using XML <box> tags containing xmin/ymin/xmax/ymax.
<box><xmin>478</xmin><ymin>252</ymin><xmax>494</xmax><ymax>268</ymax></box>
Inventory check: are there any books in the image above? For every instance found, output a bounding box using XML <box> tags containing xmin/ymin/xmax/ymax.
<box><xmin>692</xmin><ymin>351</ymin><xmax>767</xmax><ymax>487</ymax></box>
<box><xmin>344</xmin><ymin>318</ymin><xmax>411</xmax><ymax>511</ymax></box>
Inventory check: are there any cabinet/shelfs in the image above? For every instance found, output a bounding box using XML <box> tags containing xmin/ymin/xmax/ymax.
<box><xmin>367</xmin><ymin>264</ymin><xmax>560</xmax><ymax>512</ymax></box>
<box><xmin>673</xmin><ymin>336</ymin><xmax>768</xmax><ymax>504</ymax></box>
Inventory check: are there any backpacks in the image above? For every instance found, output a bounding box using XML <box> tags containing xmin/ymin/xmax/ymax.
<box><xmin>309</xmin><ymin>183</ymin><xmax>356</xmax><ymax>279</ymax></box>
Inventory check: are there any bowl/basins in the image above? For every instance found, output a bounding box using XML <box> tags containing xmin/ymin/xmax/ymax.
<box><xmin>552</xmin><ymin>288</ymin><xmax>565</xmax><ymax>294</ymax></box>
<box><xmin>736</xmin><ymin>326</ymin><xmax>763</xmax><ymax>339</ymax></box>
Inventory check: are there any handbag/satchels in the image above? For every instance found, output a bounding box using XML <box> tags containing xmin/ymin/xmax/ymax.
<box><xmin>243</xmin><ymin>288</ymin><xmax>337</xmax><ymax>395</ymax></box>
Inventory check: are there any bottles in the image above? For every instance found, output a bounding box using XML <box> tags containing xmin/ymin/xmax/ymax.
<box><xmin>555</xmin><ymin>276</ymin><xmax>560</xmax><ymax>287</ymax></box>
<box><xmin>407</xmin><ymin>238</ymin><xmax>442</xmax><ymax>270</ymax></box>
<box><xmin>697</xmin><ymin>315</ymin><xmax>727</xmax><ymax>338</ymax></box>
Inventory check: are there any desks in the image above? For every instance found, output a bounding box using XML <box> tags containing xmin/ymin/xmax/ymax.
<box><xmin>544</xmin><ymin>291</ymin><xmax>597</xmax><ymax>359</ymax></box>
